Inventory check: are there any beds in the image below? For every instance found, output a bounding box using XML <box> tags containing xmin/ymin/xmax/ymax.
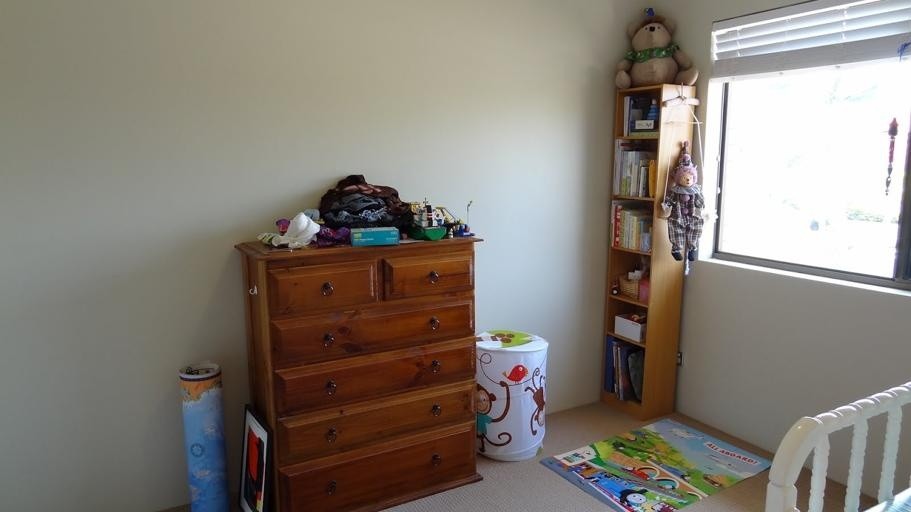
<box><xmin>766</xmin><ymin>382</ymin><xmax>911</xmax><ymax>511</ymax></box>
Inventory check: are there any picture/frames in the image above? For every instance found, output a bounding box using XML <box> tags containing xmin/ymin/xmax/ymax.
<box><xmin>239</xmin><ymin>403</ymin><xmax>272</xmax><ymax>512</ymax></box>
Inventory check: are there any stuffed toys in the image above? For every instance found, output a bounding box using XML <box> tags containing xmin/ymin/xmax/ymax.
<box><xmin>610</xmin><ymin>6</ymin><xmax>700</xmax><ymax>89</ymax></box>
<box><xmin>662</xmin><ymin>140</ymin><xmax>707</xmax><ymax>262</ymax></box>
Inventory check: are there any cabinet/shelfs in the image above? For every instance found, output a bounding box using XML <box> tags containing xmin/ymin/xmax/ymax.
<box><xmin>234</xmin><ymin>236</ymin><xmax>483</xmax><ymax>512</ymax></box>
<box><xmin>601</xmin><ymin>84</ymin><xmax>696</xmax><ymax>420</ymax></box>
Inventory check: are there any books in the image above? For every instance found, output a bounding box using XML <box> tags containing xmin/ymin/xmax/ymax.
<box><xmin>600</xmin><ymin>93</ymin><xmax>661</xmax><ymax>404</ymax></box>
